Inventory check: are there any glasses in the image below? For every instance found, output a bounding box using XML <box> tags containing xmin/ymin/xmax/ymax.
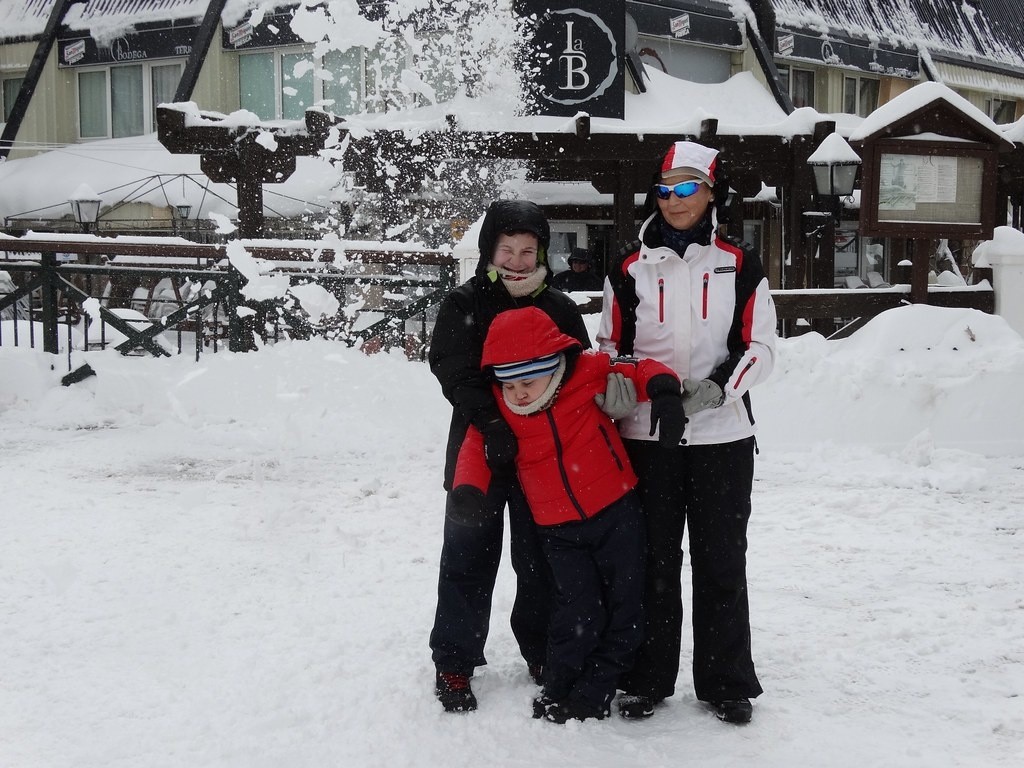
<box><xmin>652</xmin><ymin>179</ymin><xmax>705</xmax><ymax>200</ymax></box>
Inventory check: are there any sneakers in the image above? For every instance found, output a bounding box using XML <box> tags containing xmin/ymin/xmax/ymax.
<box><xmin>527</xmin><ymin>663</ymin><xmax>545</xmax><ymax>685</ymax></box>
<box><xmin>711</xmin><ymin>698</ymin><xmax>753</xmax><ymax>722</ymax></box>
<box><xmin>619</xmin><ymin>693</ymin><xmax>665</xmax><ymax>720</ymax></box>
<box><xmin>436</xmin><ymin>671</ymin><xmax>478</xmax><ymax>712</ymax></box>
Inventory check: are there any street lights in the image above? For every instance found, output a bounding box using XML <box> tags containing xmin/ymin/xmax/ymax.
<box><xmin>68</xmin><ymin>185</ymin><xmax>103</xmax><ymax>298</ymax></box>
<box><xmin>807</xmin><ymin>132</ymin><xmax>863</xmax><ymax>288</ymax></box>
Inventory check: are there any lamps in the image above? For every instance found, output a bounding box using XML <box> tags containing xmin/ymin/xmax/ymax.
<box><xmin>176</xmin><ymin>198</ymin><xmax>192</xmax><ymax>218</ymax></box>
<box><xmin>67</xmin><ymin>183</ymin><xmax>103</xmax><ymax>234</ymax></box>
<box><xmin>807</xmin><ymin>133</ymin><xmax>862</xmax><ymax>228</ymax></box>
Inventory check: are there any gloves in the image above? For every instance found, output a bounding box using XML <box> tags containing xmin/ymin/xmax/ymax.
<box><xmin>447</xmin><ymin>485</ymin><xmax>492</xmax><ymax>528</ymax></box>
<box><xmin>481</xmin><ymin>419</ymin><xmax>519</xmax><ymax>475</ymax></box>
<box><xmin>595</xmin><ymin>372</ymin><xmax>637</xmax><ymax>420</ymax></box>
<box><xmin>649</xmin><ymin>378</ymin><xmax>685</xmax><ymax>448</ymax></box>
<box><xmin>682</xmin><ymin>379</ymin><xmax>723</xmax><ymax>416</ymax></box>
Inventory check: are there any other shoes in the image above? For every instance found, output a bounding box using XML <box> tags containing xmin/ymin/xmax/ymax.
<box><xmin>532</xmin><ymin>681</ymin><xmax>611</xmax><ymax>725</ymax></box>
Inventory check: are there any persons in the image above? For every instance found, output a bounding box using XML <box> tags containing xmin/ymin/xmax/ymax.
<box><xmin>428</xmin><ymin>201</ymin><xmax>592</xmax><ymax>714</ymax></box>
<box><xmin>552</xmin><ymin>249</ymin><xmax>602</xmax><ymax>292</ymax></box>
<box><xmin>454</xmin><ymin>311</ymin><xmax>685</xmax><ymax>725</ymax></box>
<box><xmin>605</xmin><ymin>140</ymin><xmax>778</xmax><ymax>729</ymax></box>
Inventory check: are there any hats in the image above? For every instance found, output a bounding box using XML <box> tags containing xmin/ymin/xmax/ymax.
<box><xmin>493</xmin><ymin>351</ymin><xmax>560</xmax><ymax>382</ymax></box>
